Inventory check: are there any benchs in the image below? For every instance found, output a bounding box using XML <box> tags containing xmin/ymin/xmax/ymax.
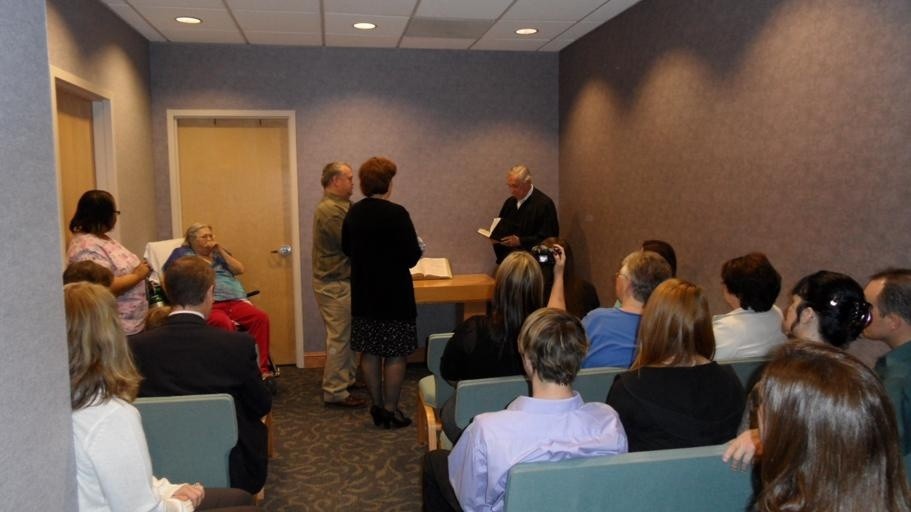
<box><xmin>133</xmin><ymin>392</ymin><xmax>239</xmax><ymax>492</ymax></box>
<box><xmin>419</xmin><ymin>334</ymin><xmax>451</xmax><ymax>405</ymax></box>
<box><xmin>500</xmin><ymin>439</ymin><xmax>765</xmax><ymax>510</ymax></box>
<box><xmin>438</xmin><ymin>357</ymin><xmax>775</xmax><ymax>451</ymax></box>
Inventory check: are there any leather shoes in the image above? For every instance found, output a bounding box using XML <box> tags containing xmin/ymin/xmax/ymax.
<box><xmin>348</xmin><ymin>378</ymin><xmax>367</xmax><ymax>389</ymax></box>
<box><xmin>327</xmin><ymin>391</ymin><xmax>370</xmax><ymax>408</ymax></box>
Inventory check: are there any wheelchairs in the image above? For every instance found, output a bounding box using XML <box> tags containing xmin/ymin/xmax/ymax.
<box><xmin>143</xmin><ymin>238</ymin><xmax>280</xmax><ymax>397</ymax></box>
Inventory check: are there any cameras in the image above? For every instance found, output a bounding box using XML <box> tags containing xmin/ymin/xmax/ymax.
<box><xmin>535</xmin><ymin>249</ymin><xmax>555</xmax><ymax>265</ymax></box>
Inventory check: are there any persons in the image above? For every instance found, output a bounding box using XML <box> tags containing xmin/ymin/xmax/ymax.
<box><xmin>68</xmin><ymin>189</ymin><xmax>154</xmax><ymax>335</ymax></box>
<box><xmin>421</xmin><ymin>236</ymin><xmax>910</xmax><ymax>512</ymax></box>
<box><xmin>62</xmin><ymin>260</ymin><xmax>114</xmax><ymax>289</ymax></box>
<box><xmin>311</xmin><ymin>160</ymin><xmax>365</xmax><ymax>408</ymax></box>
<box><xmin>163</xmin><ymin>222</ymin><xmax>278</xmax><ymax>379</ymax></box>
<box><xmin>65</xmin><ymin>281</ymin><xmax>252</xmax><ymax>511</ymax></box>
<box><xmin>125</xmin><ymin>254</ymin><xmax>274</xmax><ymax>495</ymax></box>
<box><xmin>470</xmin><ymin>164</ymin><xmax>560</xmax><ymax>264</ymax></box>
<box><xmin>340</xmin><ymin>156</ymin><xmax>423</xmax><ymax>431</ymax></box>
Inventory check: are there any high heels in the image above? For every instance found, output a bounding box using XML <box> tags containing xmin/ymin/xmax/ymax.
<box><xmin>383</xmin><ymin>407</ymin><xmax>411</xmax><ymax>429</ymax></box>
<box><xmin>370</xmin><ymin>405</ymin><xmax>382</xmax><ymax>425</ymax></box>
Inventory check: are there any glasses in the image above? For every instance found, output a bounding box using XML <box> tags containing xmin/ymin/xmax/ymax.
<box><xmin>617</xmin><ymin>271</ymin><xmax>626</xmax><ymax>277</ymax></box>
<box><xmin>113</xmin><ymin>211</ymin><xmax>119</xmax><ymax>215</ymax></box>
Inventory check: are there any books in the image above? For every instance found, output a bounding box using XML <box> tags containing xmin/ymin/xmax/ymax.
<box><xmin>477</xmin><ymin>217</ymin><xmax>505</xmax><ymax>239</ymax></box>
<box><xmin>408</xmin><ymin>257</ymin><xmax>453</xmax><ymax>280</ymax></box>
